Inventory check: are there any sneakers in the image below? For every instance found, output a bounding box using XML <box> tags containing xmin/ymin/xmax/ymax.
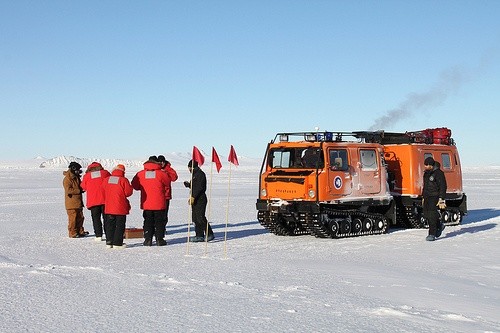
<box><xmin>426</xmin><ymin>235</ymin><xmax>435</xmax><ymax>241</ymax></box>
<box><xmin>436</xmin><ymin>224</ymin><xmax>445</xmax><ymax>237</ymax></box>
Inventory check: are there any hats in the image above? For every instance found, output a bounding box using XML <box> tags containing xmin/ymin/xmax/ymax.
<box><xmin>158</xmin><ymin>155</ymin><xmax>166</xmax><ymax>162</ymax></box>
<box><xmin>149</xmin><ymin>156</ymin><xmax>159</xmax><ymax>162</ymax></box>
<box><xmin>435</xmin><ymin>161</ymin><xmax>440</xmax><ymax>167</ymax></box>
<box><xmin>68</xmin><ymin>162</ymin><xmax>82</xmax><ymax>169</ymax></box>
<box><xmin>114</xmin><ymin>164</ymin><xmax>125</xmax><ymax>172</ymax></box>
<box><xmin>424</xmin><ymin>157</ymin><xmax>435</xmax><ymax>166</ymax></box>
<box><xmin>188</xmin><ymin>160</ymin><xmax>198</xmax><ymax>167</ymax></box>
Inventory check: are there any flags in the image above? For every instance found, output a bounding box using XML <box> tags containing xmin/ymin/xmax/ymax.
<box><xmin>192</xmin><ymin>147</ymin><xmax>206</xmax><ymax>166</ymax></box>
<box><xmin>227</xmin><ymin>146</ymin><xmax>240</xmax><ymax>167</ymax></box>
<box><xmin>212</xmin><ymin>147</ymin><xmax>223</xmax><ymax>173</ymax></box>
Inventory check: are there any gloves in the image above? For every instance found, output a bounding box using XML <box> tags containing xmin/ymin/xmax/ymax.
<box><xmin>188</xmin><ymin>197</ymin><xmax>195</xmax><ymax>205</ymax></box>
<box><xmin>184</xmin><ymin>181</ymin><xmax>190</xmax><ymax>188</ymax></box>
<box><xmin>436</xmin><ymin>200</ymin><xmax>447</xmax><ymax>209</ymax></box>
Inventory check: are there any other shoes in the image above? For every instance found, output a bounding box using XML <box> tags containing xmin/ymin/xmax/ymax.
<box><xmin>143</xmin><ymin>239</ymin><xmax>152</xmax><ymax>246</ymax></box>
<box><xmin>204</xmin><ymin>234</ymin><xmax>215</xmax><ymax>241</ymax></box>
<box><xmin>106</xmin><ymin>241</ymin><xmax>113</xmax><ymax>249</ymax></box>
<box><xmin>157</xmin><ymin>239</ymin><xmax>167</xmax><ymax>246</ymax></box>
<box><xmin>190</xmin><ymin>237</ymin><xmax>204</xmax><ymax>242</ymax></box>
<box><xmin>78</xmin><ymin>231</ymin><xmax>89</xmax><ymax>236</ymax></box>
<box><xmin>72</xmin><ymin>234</ymin><xmax>83</xmax><ymax>238</ymax></box>
<box><xmin>95</xmin><ymin>236</ymin><xmax>102</xmax><ymax>242</ymax></box>
<box><xmin>113</xmin><ymin>243</ymin><xmax>126</xmax><ymax>249</ymax></box>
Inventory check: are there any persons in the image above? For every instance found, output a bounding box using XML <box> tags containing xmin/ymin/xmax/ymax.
<box><xmin>131</xmin><ymin>156</ymin><xmax>167</xmax><ymax>246</ymax></box>
<box><xmin>184</xmin><ymin>159</ymin><xmax>216</xmax><ymax>241</ymax></box>
<box><xmin>102</xmin><ymin>164</ymin><xmax>133</xmax><ymax>248</ymax></box>
<box><xmin>152</xmin><ymin>155</ymin><xmax>178</xmax><ymax>236</ymax></box>
<box><xmin>80</xmin><ymin>162</ymin><xmax>111</xmax><ymax>241</ymax></box>
<box><xmin>421</xmin><ymin>156</ymin><xmax>446</xmax><ymax>241</ymax></box>
<box><xmin>61</xmin><ymin>161</ymin><xmax>89</xmax><ymax>238</ymax></box>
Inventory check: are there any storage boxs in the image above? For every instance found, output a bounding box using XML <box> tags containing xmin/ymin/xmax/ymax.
<box><xmin>124</xmin><ymin>228</ymin><xmax>144</xmax><ymax>239</ymax></box>
<box><xmin>303</xmin><ymin>131</ymin><xmax>334</xmax><ymax>141</ymax></box>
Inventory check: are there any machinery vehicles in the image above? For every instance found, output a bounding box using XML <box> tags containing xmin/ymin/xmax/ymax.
<box><xmin>255</xmin><ymin>127</ymin><xmax>468</xmax><ymax>239</ymax></box>
